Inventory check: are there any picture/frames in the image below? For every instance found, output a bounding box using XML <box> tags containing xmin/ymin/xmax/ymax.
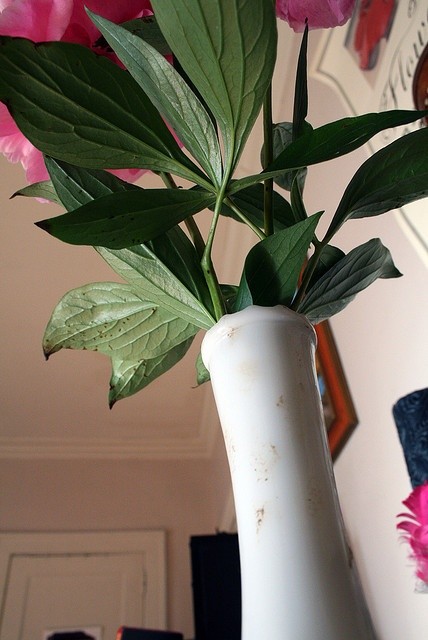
<box><xmin>313</xmin><ymin>317</ymin><xmax>357</xmax><ymax>462</ymax></box>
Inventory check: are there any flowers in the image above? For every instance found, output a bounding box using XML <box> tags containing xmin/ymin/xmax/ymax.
<box><xmin>2</xmin><ymin>1</ymin><xmax>427</xmax><ymax>405</ymax></box>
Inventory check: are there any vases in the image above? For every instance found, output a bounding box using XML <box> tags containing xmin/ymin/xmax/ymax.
<box><xmin>201</xmin><ymin>303</ymin><xmax>378</xmax><ymax>640</ymax></box>
